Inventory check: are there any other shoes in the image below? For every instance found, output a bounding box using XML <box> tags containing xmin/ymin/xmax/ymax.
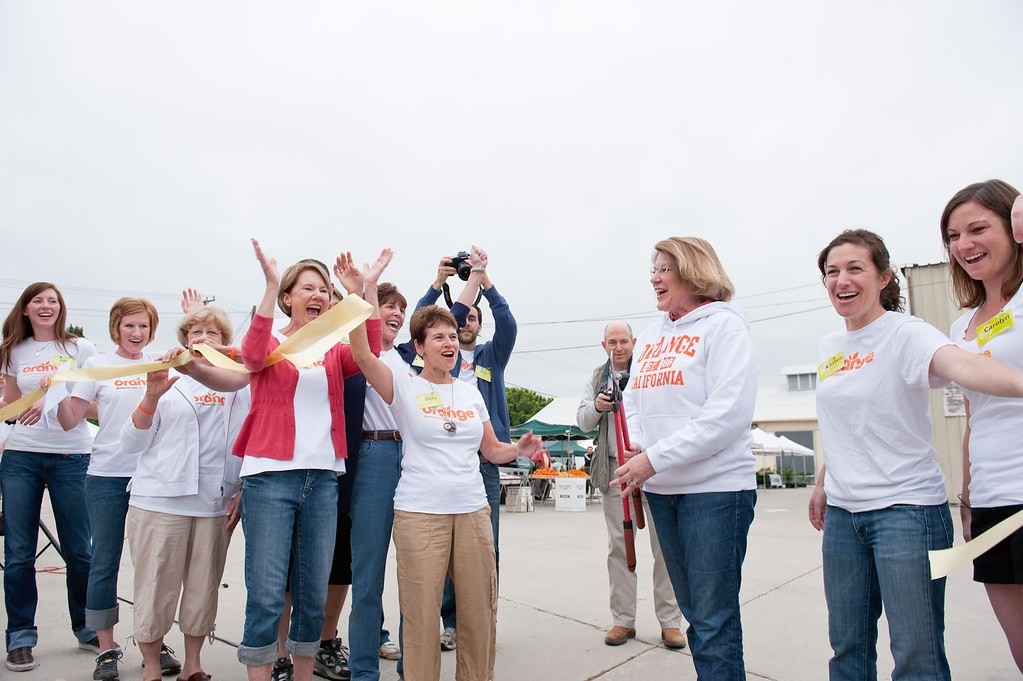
<box><xmin>6</xmin><ymin>646</ymin><xmax>35</xmax><ymax>672</ymax></box>
<box><xmin>78</xmin><ymin>634</ymin><xmax>122</xmax><ymax>655</ymax></box>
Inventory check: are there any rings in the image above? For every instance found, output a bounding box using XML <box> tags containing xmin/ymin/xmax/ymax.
<box><xmin>630</xmin><ymin>481</ymin><xmax>640</xmax><ymax>488</ymax></box>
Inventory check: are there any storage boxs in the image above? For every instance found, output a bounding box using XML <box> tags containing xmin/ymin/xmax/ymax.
<box><xmin>507</xmin><ymin>487</ymin><xmax>534</xmax><ymax>512</ymax></box>
<box><xmin>552</xmin><ymin>478</ymin><xmax>587</xmax><ymax>512</ymax></box>
<box><xmin>505</xmin><ymin>494</ymin><xmax>528</xmax><ymax>512</ymax></box>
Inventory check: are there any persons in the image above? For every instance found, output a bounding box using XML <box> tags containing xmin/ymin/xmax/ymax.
<box><xmin>534</xmin><ymin>445</ymin><xmax>551</xmax><ymax>470</ymax></box>
<box><xmin>529</xmin><ymin>458</ymin><xmax>550</xmax><ymax>501</ymax></box>
<box><xmin>809</xmin><ymin>229</ymin><xmax>1023</xmax><ymax>681</ymax></box>
<box><xmin>939</xmin><ymin>179</ymin><xmax>1023</xmax><ymax>674</ymax></box>
<box><xmin>38</xmin><ymin>296</ymin><xmax>181</xmax><ymax>681</ymax></box>
<box><xmin>576</xmin><ymin>318</ymin><xmax>686</xmax><ymax>650</ymax></box>
<box><xmin>607</xmin><ymin>236</ymin><xmax>758</xmax><ymax>681</ymax></box>
<box><xmin>0</xmin><ymin>282</ymin><xmax>120</xmax><ymax>672</ymax></box>
<box><xmin>119</xmin><ymin>235</ymin><xmax>544</xmax><ymax>681</ymax></box>
<box><xmin>583</xmin><ymin>446</ymin><xmax>596</xmax><ymax>498</ymax></box>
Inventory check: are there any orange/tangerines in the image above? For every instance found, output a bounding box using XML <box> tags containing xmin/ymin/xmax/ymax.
<box><xmin>533</xmin><ymin>467</ymin><xmax>587</xmax><ymax>477</ymax></box>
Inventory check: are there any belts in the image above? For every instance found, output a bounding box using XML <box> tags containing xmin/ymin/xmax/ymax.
<box><xmin>362</xmin><ymin>430</ymin><xmax>402</xmax><ymax>442</ymax></box>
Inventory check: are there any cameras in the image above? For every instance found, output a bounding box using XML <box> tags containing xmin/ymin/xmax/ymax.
<box><xmin>444</xmin><ymin>251</ymin><xmax>473</xmax><ymax>281</ymax></box>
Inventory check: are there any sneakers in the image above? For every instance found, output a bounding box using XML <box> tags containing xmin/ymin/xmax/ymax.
<box><xmin>661</xmin><ymin>628</ymin><xmax>686</xmax><ymax>648</ymax></box>
<box><xmin>605</xmin><ymin>625</ymin><xmax>636</xmax><ymax>646</ymax></box>
<box><xmin>438</xmin><ymin>626</ymin><xmax>457</xmax><ymax>651</ymax></box>
<box><xmin>312</xmin><ymin>629</ymin><xmax>352</xmax><ymax>681</ymax></box>
<box><xmin>92</xmin><ymin>647</ymin><xmax>124</xmax><ymax>681</ymax></box>
<box><xmin>270</xmin><ymin>652</ymin><xmax>295</xmax><ymax>681</ymax></box>
<box><xmin>378</xmin><ymin>641</ymin><xmax>401</xmax><ymax>659</ymax></box>
<box><xmin>141</xmin><ymin>643</ymin><xmax>181</xmax><ymax>675</ymax></box>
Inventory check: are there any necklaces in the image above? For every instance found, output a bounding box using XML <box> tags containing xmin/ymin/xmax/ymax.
<box><xmin>33</xmin><ymin>339</ymin><xmax>54</xmax><ymax>357</ymax></box>
<box><xmin>422</xmin><ymin>370</ymin><xmax>456</xmax><ymax>434</ymax></box>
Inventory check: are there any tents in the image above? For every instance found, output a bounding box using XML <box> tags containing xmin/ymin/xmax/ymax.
<box><xmin>776</xmin><ymin>433</ymin><xmax>814</xmax><ymax>489</ymax></box>
<box><xmin>544</xmin><ymin>438</ymin><xmax>587</xmax><ymax>471</ymax></box>
<box><xmin>746</xmin><ymin>427</ymin><xmax>785</xmax><ymax>490</ymax></box>
<box><xmin>508</xmin><ymin>418</ymin><xmax>601</xmax><ymax>475</ymax></box>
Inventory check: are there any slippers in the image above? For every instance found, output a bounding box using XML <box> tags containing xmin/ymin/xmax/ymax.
<box><xmin>176</xmin><ymin>672</ymin><xmax>212</xmax><ymax>681</ymax></box>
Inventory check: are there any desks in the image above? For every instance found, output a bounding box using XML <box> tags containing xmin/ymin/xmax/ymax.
<box><xmin>528</xmin><ymin>476</ymin><xmax>593</xmax><ymax>508</ymax></box>
<box><xmin>500</xmin><ymin>478</ymin><xmax>528</xmax><ymax>513</ymax></box>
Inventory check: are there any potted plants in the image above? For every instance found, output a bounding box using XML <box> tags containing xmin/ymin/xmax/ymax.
<box><xmin>756</xmin><ymin>465</ymin><xmax>811</xmax><ymax>488</ymax></box>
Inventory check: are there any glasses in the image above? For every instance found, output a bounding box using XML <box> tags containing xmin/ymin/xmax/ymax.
<box><xmin>651</xmin><ymin>263</ymin><xmax>678</xmax><ymax>277</ymax></box>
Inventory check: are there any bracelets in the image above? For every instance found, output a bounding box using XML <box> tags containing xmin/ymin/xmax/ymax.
<box><xmin>340</xmin><ymin>269</ymin><xmax>344</xmax><ymax>272</ymax></box>
<box><xmin>470</xmin><ymin>267</ymin><xmax>486</xmax><ymax>274</ymax></box>
<box><xmin>231</xmin><ymin>345</ymin><xmax>236</xmax><ymax>359</ymax></box>
<box><xmin>139</xmin><ymin>403</ymin><xmax>155</xmax><ymax>415</ymax></box>
<box><xmin>514</xmin><ymin>444</ymin><xmax>520</xmax><ymax>460</ymax></box>
